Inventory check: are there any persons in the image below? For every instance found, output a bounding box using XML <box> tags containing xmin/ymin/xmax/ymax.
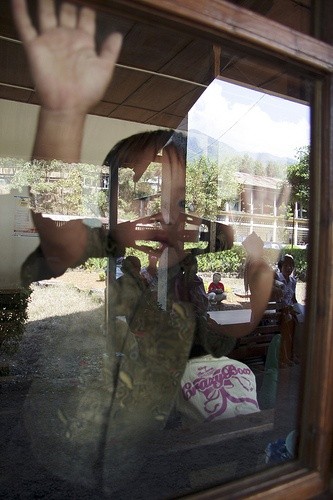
<box><xmin>262</xmin><ymin>307</ymin><xmax>296</xmax><ymax>409</ymax></box>
<box><xmin>0</xmin><ymin>1</ymin><xmax>292</xmax><ymax>499</ymax></box>
<box><xmin>181</xmin><ymin>326</ymin><xmax>261</xmax><ymax>424</ymax></box>
<box><xmin>272</xmin><ymin>254</ymin><xmax>306</xmax><ymax>366</ymax></box>
<box><xmin>141</xmin><ymin>249</ymin><xmax>158</xmax><ymax>298</ymax></box>
<box><xmin>207</xmin><ymin>273</ymin><xmax>226</xmax><ymax>305</ymax></box>
<box><xmin>106</xmin><ymin>256</ymin><xmax>168</xmax><ymax>334</ymax></box>
<box><xmin>241</xmin><ymin>231</ymin><xmax>296</xmax><ymax>368</ymax></box>
<box><xmin>174</xmin><ymin>253</ymin><xmax>209</xmax><ymax>357</ymax></box>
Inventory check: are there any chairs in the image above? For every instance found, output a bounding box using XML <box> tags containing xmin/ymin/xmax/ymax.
<box><xmin>238</xmin><ymin>287</ymin><xmax>297</xmax><ymax>361</ymax></box>
<box><xmin>0</xmin><ymin>407</ymin><xmax>275</xmax><ymax>500</ymax></box>
